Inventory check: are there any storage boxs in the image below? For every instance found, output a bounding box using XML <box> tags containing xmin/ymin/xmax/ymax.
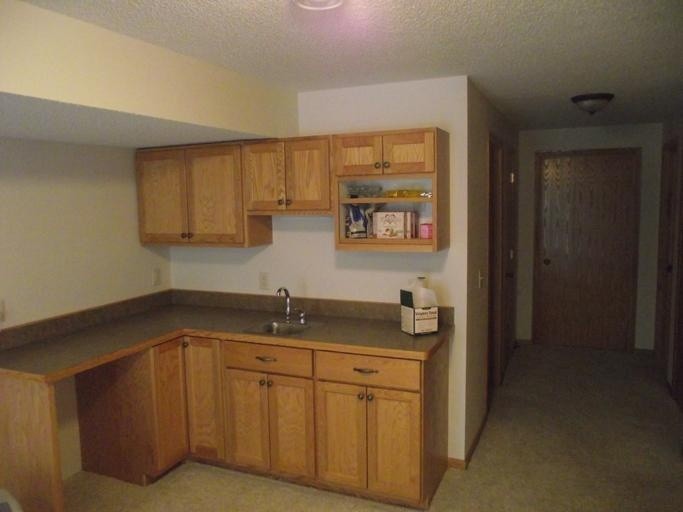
<box><xmin>398</xmin><ymin>288</ymin><xmax>440</xmax><ymax>337</ymax></box>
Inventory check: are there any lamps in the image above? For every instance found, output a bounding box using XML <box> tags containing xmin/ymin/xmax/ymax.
<box><xmin>567</xmin><ymin>92</ymin><xmax>615</xmax><ymax>118</ymax></box>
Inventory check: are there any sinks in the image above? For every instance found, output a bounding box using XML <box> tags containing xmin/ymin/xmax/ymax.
<box><xmin>242</xmin><ymin>320</ymin><xmax>313</xmax><ymax>338</ymax></box>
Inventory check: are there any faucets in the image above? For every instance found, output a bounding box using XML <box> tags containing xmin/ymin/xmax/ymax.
<box><xmin>276</xmin><ymin>287</ymin><xmax>291</xmax><ymax>323</ymax></box>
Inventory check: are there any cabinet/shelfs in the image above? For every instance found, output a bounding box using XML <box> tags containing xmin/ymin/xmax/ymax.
<box><xmin>311</xmin><ymin>330</ymin><xmax>451</xmax><ymax>510</ymax></box>
<box><xmin>240</xmin><ymin>134</ymin><xmax>333</xmax><ymax>218</ymax></box>
<box><xmin>74</xmin><ymin>334</ymin><xmax>189</xmax><ymax>487</ymax></box>
<box><xmin>180</xmin><ymin>332</ymin><xmax>221</xmax><ymax>470</ymax></box>
<box><xmin>220</xmin><ymin>338</ymin><xmax>315</xmax><ymax>491</ymax></box>
<box><xmin>132</xmin><ymin>140</ymin><xmax>273</xmax><ymax>248</ymax></box>
<box><xmin>329</xmin><ymin>125</ymin><xmax>451</xmax><ymax>254</ymax></box>
